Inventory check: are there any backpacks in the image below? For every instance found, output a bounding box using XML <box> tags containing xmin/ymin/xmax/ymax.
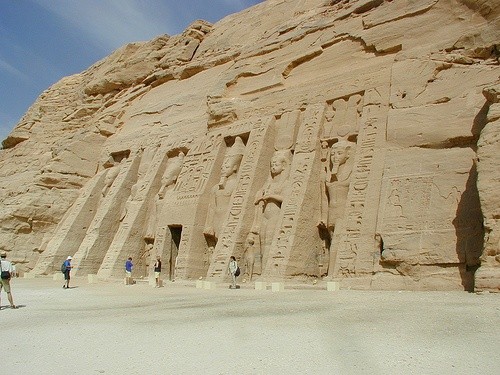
<box><xmin>60</xmin><ymin>264</ymin><xmax>67</xmax><ymax>273</ymax></box>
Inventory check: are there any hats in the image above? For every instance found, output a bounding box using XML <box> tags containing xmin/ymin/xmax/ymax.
<box><xmin>0</xmin><ymin>252</ymin><xmax>7</xmax><ymax>256</ymax></box>
<box><xmin>156</xmin><ymin>256</ymin><xmax>160</xmax><ymax>259</ymax></box>
<box><xmin>67</xmin><ymin>256</ymin><xmax>72</xmax><ymax>260</ymax></box>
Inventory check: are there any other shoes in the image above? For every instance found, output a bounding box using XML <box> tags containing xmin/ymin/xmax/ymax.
<box><xmin>67</xmin><ymin>286</ymin><xmax>69</xmax><ymax>288</ymax></box>
<box><xmin>63</xmin><ymin>285</ymin><xmax>65</xmax><ymax>287</ymax></box>
<box><xmin>0</xmin><ymin>306</ymin><xmax>6</xmax><ymax>310</ymax></box>
<box><xmin>231</xmin><ymin>286</ymin><xmax>236</xmax><ymax>289</ymax></box>
<box><xmin>11</xmin><ymin>305</ymin><xmax>18</xmax><ymax>309</ymax></box>
<box><xmin>152</xmin><ymin>286</ymin><xmax>160</xmax><ymax>288</ymax></box>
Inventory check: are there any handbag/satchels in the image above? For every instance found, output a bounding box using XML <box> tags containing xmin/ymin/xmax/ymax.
<box><xmin>1</xmin><ymin>271</ymin><xmax>10</xmax><ymax>279</ymax></box>
<box><xmin>234</xmin><ymin>261</ymin><xmax>240</xmax><ymax>276</ymax></box>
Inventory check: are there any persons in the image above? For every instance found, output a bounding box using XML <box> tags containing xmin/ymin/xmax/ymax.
<box><xmin>62</xmin><ymin>256</ymin><xmax>73</xmax><ymax>289</ymax></box>
<box><xmin>316</xmin><ymin>140</ymin><xmax>352</xmax><ymax>279</ymax></box>
<box><xmin>143</xmin><ymin>152</ymin><xmax>186</xmax><ymax>267</ymax></box>
<box><xmin>0</xmin><ymin>252</ymin><xmax>18</xmax><ymax>309</ymax></box>
<box><xmin>203</xmin><ymin>137</ymin><xmax>246</xmax><ymax>265</ymax></box>
<box><xmin>228</xmin><ymin>256</ymin><xmax>238</xmax><ymax>289</ymax></box>
<box><xmin>97</xmin><ymin>156</ymin><xmax>126</xmax><ymax>203</ymax></box>
<box><xmin>125</xmin><ymin>257</ymin><xmax>134</xmax><ymax>285</ymax></box>
<box><xmin>242</xmin><ymin>149</ymin><xmax>293</xmax><ymax>280</ymax></box>
<box><xmin>153</xmin><ymin>256</ymin><xmax>161</xmax><ymax>287</ymax></box>
